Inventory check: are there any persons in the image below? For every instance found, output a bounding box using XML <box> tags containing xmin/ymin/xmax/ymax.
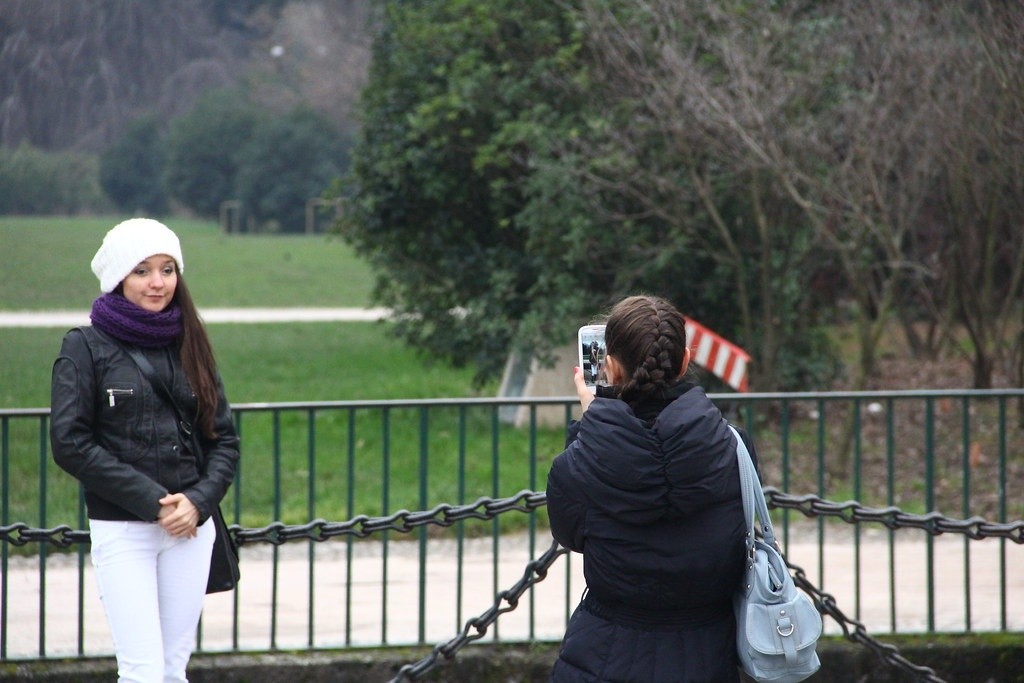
<box><xmin>547</xmin><ymin>290</ymin><xmax>764</xmax><ymax>683</ymax></box>
<box><xmin>589</xmin><ymin>340</ymin><xmax>599</xmax><ymax>383</ymax></box>
<box><xmin>49</xmin><ymin>218</ymin><xmax>241</xmax><ymax>683</ymax></box>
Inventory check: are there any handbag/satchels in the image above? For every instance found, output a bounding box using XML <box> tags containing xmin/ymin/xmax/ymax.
<box><xmin>205</xmin><ymin>505</ymin><xmax>240</xmax><ymax>594</ymax></box>
<box><xmin>728</xmin><ymin>424</ymin><xmax>822</xmax><ymax>682</ymax></box>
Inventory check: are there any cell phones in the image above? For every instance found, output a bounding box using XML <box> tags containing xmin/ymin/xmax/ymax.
<box><xmin>578</xmin><ymin>324</ymin><xmax>607</xmax><ymax>396</ymax></box>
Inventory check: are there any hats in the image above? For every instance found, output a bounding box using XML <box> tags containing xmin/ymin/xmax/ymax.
<box><xmin>91</xmin><ymin>218</ymin><xmax>183</xmax><ymax>294</ymax></box>
